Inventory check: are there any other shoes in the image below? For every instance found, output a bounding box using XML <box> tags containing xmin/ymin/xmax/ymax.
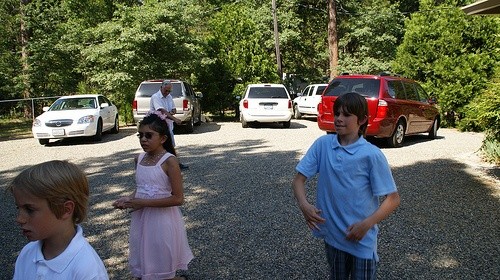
<box><xmin>179</xmin><ymin>163</ymin><xmax>189</xmax><ymax>169</ymax></box>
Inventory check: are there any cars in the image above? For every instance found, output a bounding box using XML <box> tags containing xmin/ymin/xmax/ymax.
<box><xmin>32</xmin><ymin>93</ymin><xmax>119</xmax><ymax>146</ymax></box>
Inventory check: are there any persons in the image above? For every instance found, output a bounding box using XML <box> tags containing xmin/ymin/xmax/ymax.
<box><xmin>6</xmin><ymin>159</ymin><xmax>110</xmax><ymax>280</ymax></box>
<box><xmin>112</xmin><ymin>112</ymin><xmax>194</xmax><ymax>280</ymax></box>
<box><xmin>293</xmin><ymin>93</ymin><xmax>401</xmax><ymax>280</ymax></box>
<box><xmin>149</xmin><ymin>80</ymin><xmax>190</xmax><ymax>170</ymax></box>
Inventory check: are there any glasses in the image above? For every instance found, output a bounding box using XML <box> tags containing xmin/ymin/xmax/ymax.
<box><xmin>165</xmin><ymin>89</ymin><xmax>172</xmax><ymax>93</ymax></box>
<box><xmin>137</xmin><ymin>132</ymin><xmax>155</xmax><ymax>138</ymax></box>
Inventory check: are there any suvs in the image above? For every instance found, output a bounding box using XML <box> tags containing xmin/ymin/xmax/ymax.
<box><xmin>236</xmin><ymin>84</ymin><xmax>293</xmax><ymax>128</ymax></box>
<box><xmin>132</xmin><ymin>80</ymin><xmax>203</xmax><ymax>133</ymax></box>
<box><xmin>316</xmin><ymin>72</ymin><xmax>440</xmax><ymax>148</ymax></box>
<box><xmin>292</xmin><ymin>84</ymin><xmax>346</xmax><ymax>121</ymax></box>
